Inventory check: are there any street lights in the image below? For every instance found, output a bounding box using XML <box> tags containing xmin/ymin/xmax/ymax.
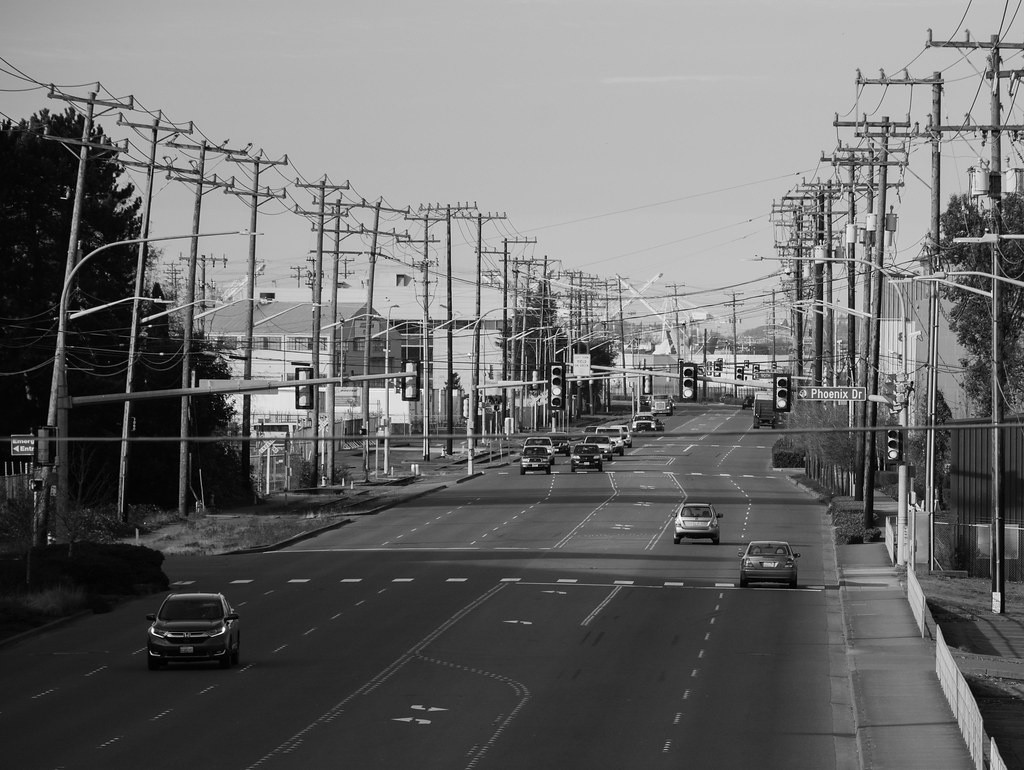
<box><xmin>61</xmin><ymin>294</ymin><xmax>622</xmax><ymax>497</ymax></box>
<box><xmin>779</xmin><ymin>297</ymin><xmax>879</xmax><ymax>488</ymax></box>
<box><xmin>750</xmin><ymin>255</ymin><xmax>914</xmax><ymax>525</ymax></box>
<box><xmin>29</xmin><ymin>227</ymin><xmax>262</xmax><ymax>544</ymax></box>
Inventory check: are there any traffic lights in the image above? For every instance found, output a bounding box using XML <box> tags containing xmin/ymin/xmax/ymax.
<box><xmin>294</xmin><ymin>367</ymin><xmax>315</xmax><ymax>410</ymax></box>
<box><xmin>642</xmin><ymin>367</ymin><xmax>653</xmax><ymax>394</ymax></box>
<box><xmin>399</xmin><ymin>358</ymin><xmax>421</xmax><ymax>401</ymax></box>
<box><xmin>679</xmin><ymin>364</ymin><xmax>698</xmax><ymax>401</ymax></box>
<box><xmin>771</xmin><ymin>373</ymin><xmax>791</xmax><ymax>412</ymax></box>
<box><xmin>885</xmin><ymin>425</ymin><xmax>905</xmax><ymax>465</ymax></box>
<box><xmin>547</xmin><ymin>363</ymin><xmax>567</xmax><ymax>411</ymax></box>
<box><xmin>678</xmin><ymin>357</ymin><xmax>777</xmax><ymax>387</ymax></box>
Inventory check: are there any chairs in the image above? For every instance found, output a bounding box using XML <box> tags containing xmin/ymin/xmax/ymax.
<box><xmin>702</xmin><ymin>511</ymin><xmax>710</xmax><ymax>517</ymax></box>
<box><xmin>683</xmin><ymin>510</ymin><xmax>693</xmax><ymax>517</ymax></box>
<box><xmin>753</xmin><ymin>546</ymin><xmax>761</xmax><ymax>555</ymax></box>
<box><xmin>775</xmin><ymin>547</ymin><xmax>786</xmax><ymax>555</ymax></box>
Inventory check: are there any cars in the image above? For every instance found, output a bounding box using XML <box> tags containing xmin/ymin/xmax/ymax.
<box><xmin>737</xmin><ymin>540</ymin><xmax>801</xmax><ymax>588</ymax></box>
<box><xmin>671</xmin><ymin>502</ymin><xmax>724</xmax><ymax>544</ymax></box>
<box><xmin>634</xmin><ymin>396</ymin><xmax>648</xmax><ymax>406</ymax></box>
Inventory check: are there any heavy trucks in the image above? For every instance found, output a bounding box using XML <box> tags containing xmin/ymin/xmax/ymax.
<box><xmin>650</xmin><ymin>393</ymin><xmax>674</xmax><ymax>415</ymax></box>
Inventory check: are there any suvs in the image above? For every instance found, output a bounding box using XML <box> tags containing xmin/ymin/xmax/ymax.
<box><xmin>146</xmin><ymin>591</ymin><xmax>240</xmax><ymax>668</ymax></box>
<box><xmin>516</xmin><ymin>413</ymin><xmax>665</xmax><ymax>474</ymax></box>
